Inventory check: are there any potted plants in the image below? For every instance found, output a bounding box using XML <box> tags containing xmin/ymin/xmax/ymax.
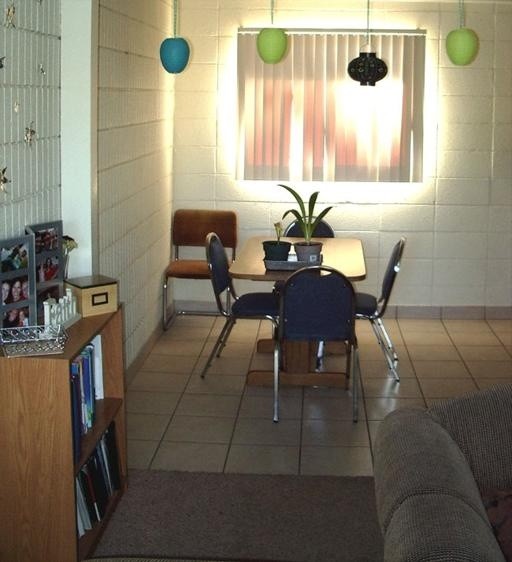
<box><xmin>262</xmin><ymin>221</ymin><xmax>291</xmax><ymax>260</ymax></box>
<box><xmin>276</xmin><ymin>183</ymin><xmax>333</xmax><ymax>263</ymax></box>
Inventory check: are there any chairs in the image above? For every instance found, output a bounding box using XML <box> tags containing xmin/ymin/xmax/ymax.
<box><xmin>263</xmin><ymin>265</ymin><xmax>360</xmax><ymax>423</ymax></box>
<box><xmin>315</xmin><ymin>235</ymin><xmax>406</xmax><ymax>382</ymax></box>
<box><xmin>284</xmin><ymin>215</ymin><xmax>335</xmax><ymax>238</ymax></box>
<box><xmin>162</xmin><ymin>209</ymin><xmax>238</xmax><ymax>332</ymax></box>
<box><xmin>199</xmin><ymin>231</ymin><xmax>282</xmax><ymax>381</ymax></box>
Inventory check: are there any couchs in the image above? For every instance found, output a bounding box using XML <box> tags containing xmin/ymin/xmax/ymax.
<box><xmin>372</xmin><ymin>382</ymin><xmax>511</xmax><ymax>561</ymax></box>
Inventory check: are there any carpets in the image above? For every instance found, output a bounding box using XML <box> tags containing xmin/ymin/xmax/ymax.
<box><xmin>86</xmin><ymin>467</ymin><xmax>386</xmax><ymax>562</ymax></box>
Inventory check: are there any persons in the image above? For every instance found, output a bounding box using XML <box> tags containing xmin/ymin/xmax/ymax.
<box><xmin>0</xmin><ymin>226</ymin><xmax>59</xmax><ymax>334</ymax></box>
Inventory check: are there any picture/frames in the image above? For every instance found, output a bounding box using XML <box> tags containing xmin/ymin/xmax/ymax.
<box><xmin>0</xmin><ymin>233</ymin><xmax>37</xmax><ymax>329</ymax></box>
<box><xmin>26</xmin><ymin>219</ymin><xmax>66</xmax><ymax>320</ymax></box>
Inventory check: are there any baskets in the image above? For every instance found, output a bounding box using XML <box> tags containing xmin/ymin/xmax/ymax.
<box><xmin>0</xmin><ymin>324</ymin><xmax>68</xmax><ymax>358</ymax></box>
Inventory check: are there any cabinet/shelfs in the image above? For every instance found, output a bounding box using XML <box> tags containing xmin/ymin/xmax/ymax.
<box><xmin>0</xmin><ymin>300</ymin><xmax>128</xmax><ymax>561</ymax></box>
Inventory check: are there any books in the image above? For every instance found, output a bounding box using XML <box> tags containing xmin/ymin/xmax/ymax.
<box><xmin>70</xmin><ymin>344</ymin><xmax>119</xmax><ymax>539</ymax></box>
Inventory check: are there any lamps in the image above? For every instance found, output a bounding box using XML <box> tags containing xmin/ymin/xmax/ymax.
<box><xmin>158</xmin><ymin>0</ymin><xmax>191</xmax><ymax>74</ymax></box>
<box><xmin>257</xmin><ymin>0</ymin><xmax>288</xmax><ymax>65</ymax></box>
<box><xmin>446</xmin><ymin>0</ymin><xmax>480</xmax><ymax>66</ymax></box>
<box><xmin>346</xmin><ymin>1</ymin><xmax>389</xmax><ymax>87</ymax></box>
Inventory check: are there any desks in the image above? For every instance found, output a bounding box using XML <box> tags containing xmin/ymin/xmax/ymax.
<box><xmin>226</xmin><ymin>234</ymin><xmax>367</xmax><ymax>387</ymax></box>
